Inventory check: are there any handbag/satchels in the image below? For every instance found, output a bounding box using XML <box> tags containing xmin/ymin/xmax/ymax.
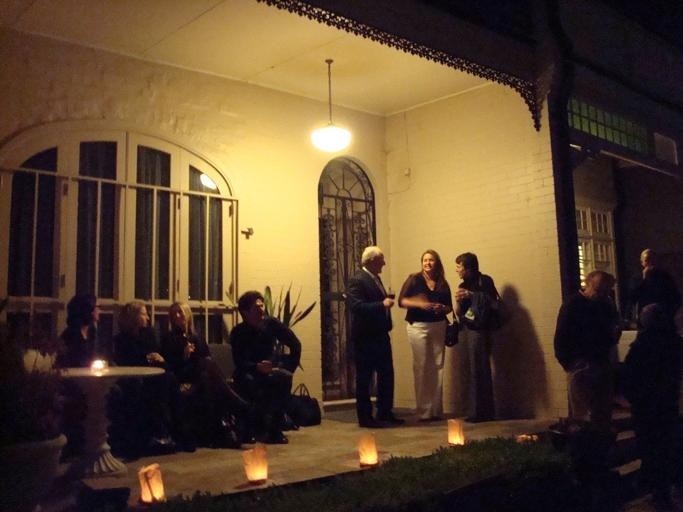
<box><xmin>444</xmin><ymin>307</ymin><xmax>460</xmax><ymax>347</ymax></box>
<box><xmin>287</xmin><ymin>383</ymin><xmax>321</xmax><ymax>426</ymax></box>
<box><xmin>486</xmin><ymin>285</ymin><xmax>511</xmax><ymax>330</ymax></box>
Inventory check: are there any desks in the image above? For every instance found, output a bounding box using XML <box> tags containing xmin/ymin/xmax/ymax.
<box><xmin>57</xmin><ymin>365</ymin><xmax>165</xmax><ymax>478</ymax></box>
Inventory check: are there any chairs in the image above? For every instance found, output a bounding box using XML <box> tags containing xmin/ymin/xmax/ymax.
<box><xmin>207</xmin><ymin>342</ymin><xmax>239</xmax><ymax>385</ymax></box>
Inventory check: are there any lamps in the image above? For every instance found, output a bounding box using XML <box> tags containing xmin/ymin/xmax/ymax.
<box><xmin>137</xmin><ymin>463</ymin><xmax>165</xmax><ymax>505</ymax></box>
<box><xmin>445</xmin><ymin>418</ymin><xmax>464</xmax><ymax>447</ymax></box>
<box><xmin>241</xmin><ymin>445</ymin><xmax>268</xmax><ymax>486</ymax></box>
<box><xmin>357</xmin><ymin>430</ymin><xmax>379</xmax><ymax>468</ymax></box>
<box><xmin>308</xmin><ymin>59</ymin><xmax>352</xmax><ymax>153</ymax></box>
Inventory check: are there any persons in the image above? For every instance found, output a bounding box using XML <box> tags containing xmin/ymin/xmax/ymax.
<box><xmin>56</xmin><ymin>291</ymin><xmax>112</xmax><ymax>368</ymax></box>
<box><xmin>552</xmin><ymin>269</ymin><xmax>622</xmax><ymax>428</ymax></box>
<box><xmin>630</xmin><ymin>249</ymin><xmax>676</xmax><ymax>309</ymax></box>
<box><xmin>344</xmin><ymin>245</ymin><xmax>404</xmax><ymax>429</ymax></box>
<box><xmin>453</xmin><ymin>254</ymin><xmax>505</xmax><ymax>422</ymax></box>
<box><xmin>107</xmin><ymin>302</ymin><xmax>176</xmax><ymax>455</ymax></box>
<box><xmin>231</xmin><ymin>290</ymin><xmax>302</xmax><ymax>444</ymax></box>
<box><xmin>611</xmin><ymin>304</ymin><xmax>683</xmax><ymax>512</ymax></box>
<box><xmin>162</xmin><ymin>302</ymin><xmax>252</xmax><ymax>448</ymax></box>
<box><xmin>398</xmin><ymin>250</ymin><xmax>453</xmax><ymax>422</ymax></box>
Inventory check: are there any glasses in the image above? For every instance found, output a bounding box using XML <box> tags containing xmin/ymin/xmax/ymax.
<box><xmin>141</xmin><ymin>312</ymin><xmax>147</xmax><ymax>315</ymax></box>
<box><xmin>95</xmin><ymin>305</ymin><xmax>102</xmax><ymax>309</ymax></box>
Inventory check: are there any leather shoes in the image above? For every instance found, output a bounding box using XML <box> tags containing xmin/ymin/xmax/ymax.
<box><xmin>376</xmin><ymin>409</ymin><xmax>405</xmax><ymax>425</ymax></box>
<box><xmin>360</xmin><ymin>418</ymin><xmax>393</xmax><ymax>428</ymax></box>
